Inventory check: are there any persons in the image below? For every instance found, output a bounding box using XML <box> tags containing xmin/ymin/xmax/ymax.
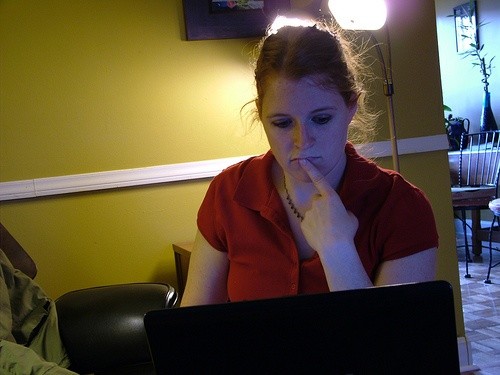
<box><xmin>180</xmin><ymin>7</ymin><xmax>439</xmax><ymax>306</ymax></box>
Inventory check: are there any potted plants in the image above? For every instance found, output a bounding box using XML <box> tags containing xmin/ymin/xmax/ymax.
<box><xmin>447</xmin><ymin>0</ymin><xmax>499</xmax><ymax>144</ymax></box>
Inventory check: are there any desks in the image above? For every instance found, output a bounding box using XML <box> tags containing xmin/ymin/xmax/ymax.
<box><xmin>448</xmin><ymin>144</ymin><xmax>500</xmax><ymax>263</ymax></box>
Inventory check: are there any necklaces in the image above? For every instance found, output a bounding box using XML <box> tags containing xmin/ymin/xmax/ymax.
<box><xmin>284</xmin><ymin>175</ymin><xmax>304</xmax><ymax>220</ymax></box>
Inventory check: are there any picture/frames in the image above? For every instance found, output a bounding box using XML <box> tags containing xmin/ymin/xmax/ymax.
<box><xmin>454</xmin><ymin>0</ymin><xmax>480</xmax><ymax>52</ymax></box>
<box><xmin>182</xmin><ymin>0</ymin><xmax>290</xmax><ymax>41</ymax></box>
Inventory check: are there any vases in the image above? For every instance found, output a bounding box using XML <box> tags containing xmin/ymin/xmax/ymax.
<box><xmin>480</xmin><ymin>91</ymin><xmax>498</xmax><ymax>133</ymax></box>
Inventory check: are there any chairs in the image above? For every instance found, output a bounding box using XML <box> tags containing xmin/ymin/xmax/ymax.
<box><xmin>450</xmin><ymin>130</ymin><xmax>500</xmax><ymax>284</ymax></box>
<box><xmin>54</xmin><ymin>283</ymin><xmax>179</xmax><ymax>375</ymax></box>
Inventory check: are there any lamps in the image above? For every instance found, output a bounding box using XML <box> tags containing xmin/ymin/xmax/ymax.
<box><xmin>263</xmin><ymin>0</ymin><xmax>399</xmax><ymax>175</ymax></box>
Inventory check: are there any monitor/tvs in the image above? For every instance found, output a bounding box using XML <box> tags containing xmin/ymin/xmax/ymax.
<box><xmin>143</xmin><ymin>280</ymin><xmax>461</xmax><ymax>375</ymax></box>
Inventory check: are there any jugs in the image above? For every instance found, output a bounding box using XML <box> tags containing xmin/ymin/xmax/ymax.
<box><xmin>447</xmin><ymin>117</ymin><xmax>470</xmax><ymax>150</ymax></box>
